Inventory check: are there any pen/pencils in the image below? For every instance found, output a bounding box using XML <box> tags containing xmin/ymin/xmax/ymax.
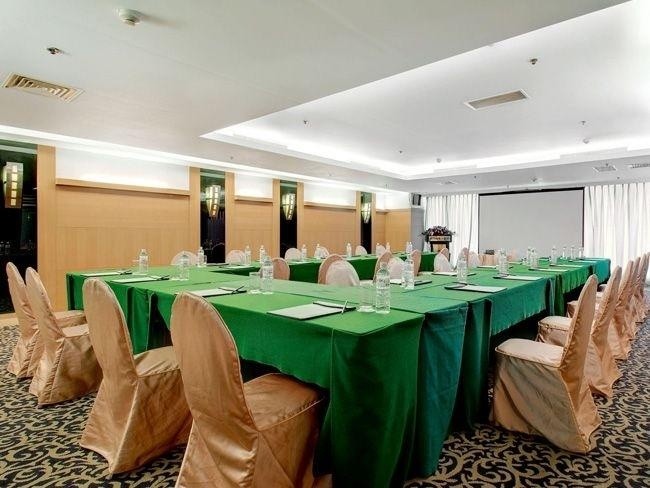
<box><xmin>121</xmin><ymin>270</ymin><xmax>132</xmax><ymax>274</ymax></box>
<box><xmin>158</xmin><ymin>274</ymin><xmax>170</xmax><ymax>280</ymax></box>
<box><xmin>231</xmin><ymin>285</ymin><xmax>244</xmax><ymax>294</ymax></box>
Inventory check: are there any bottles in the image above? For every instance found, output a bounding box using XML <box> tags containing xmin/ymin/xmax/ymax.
<box><xmin>459</xmin><ymin>258</ymin><xmax>467</xmax><ymax>282</ymax></box>
<box><xmin>376</xmin><ymin>243</ymin><xmax>380</xmax><ymax>257</ymax></box>
<box><xmin>526</xmin><ymin>249</ymin><xmax>531</xmax><ymax>266</ymax></box>
<box><xmin>316</xmin><ymin>243</ymin><xmax>321</xmax><ymax>259</ymax></box>
<box><xmin>530</xmin><ymin>250</ymin><xmax>537</xmax><ymax>269</ymax></box>
<box><xmin>579</xmin><ymin>245</ymin><xmax>584</xmax><ymax>259</ymax></box>
<box><xmin>404</xmin><ymin>255</ymin><xmax>414</xmax><ymax>290</ymax></box>
<box><xmin>570</xmin><ymin>245</ymin><xmax>575</xmax><ymax>260</ymax></box>
<box><xmin>376</xmin><ymin>262</ymin><xmax>391</xmax><ymax>314</ymax></box>
<box><xmin>262</xmin><ymin>256</ymin><xmax>274</xmax><ymax>294</ymax></box>
<box><xmin>552</xmin><ymin>246</ymin><xmax>557</xmax><ymax>264</ymax></box>
<box><xmin>495</xmin><ymin>250</ymin><xmax>501</xmax><ymax>270</ymax></box>
<box><xmin>196</xmin><ymin>247</ymin><xmax>204</xmax><ymax>268</ymax></box>
<box><xmin>245</xmin><ymin>246</ymin><xmax>251</xmax><ymax>265</ymax></box>
<box><xmin>427</xmin><ymin>242</ymin><xmax>431</xmax><ymax>253</ymax></box>
<box><xmin>301</xmin><ymin>244</ymin><xmax>307</xmax><ymax>261</ymax></box>
<box><xmin>180</xmin><ymin>250</ymin><xmax>189</xmax><ymax>281</ymax></box>
<box><xmin>500</xmin><ymin>253</ymin><xmax>508</xmax><ymax>276</ymax></box>
<box><xmin>562</xmin><ymin>245</ymin><xmax>567</xmax><ymax>260</ymax></box>
<box><xmin>347</xmin><ymin>243</ymin><xmax>352</xmax><ymax>258</ymax></box>
<box><xmin>408</xmin><ymin>242</ymin><xmax>412</xmax><ymax>253</ymax></box>
<box><xmin>140</xmin><ymin>249</ymin><xmax>148</xmax><ymax>273</ymax></box>
<box><xmin>260</xmin><ymin>245</ymin><xmax>265</xmax><ymax>263</ymax></box>
<box><xmin>386</xmin><ymin>242</ymin><xmax>390</xmax><ymax>251</ymax></box>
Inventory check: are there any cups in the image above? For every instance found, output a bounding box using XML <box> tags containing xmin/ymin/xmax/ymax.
<box><xmin>357</xmin><ymin>282</ymin><xmax>375</xmax><ymax>312</ymax></box>
<box><xmin>240</xmin><ymin>256</ymin><xmax>245</xmax><ymax>265</ymax></box>
<box><xmin>520</xmin><ymin>257</ymin><xmax>526</xmax><ymax>266</ymax></box>
<box><xmin>560</xmin><ymin>254</ymin><xmax>563</xmax><ymax>259</ymax></box>
<box><xmin>204</xmin><ymin>255</ymin><xmax>207</xmax><ymax>267</ymax></box>
<box><xmin>549</xmin><ymin>256</ymin><xmax>551</xmax><ymax>262</ymax></box>
<box><xmin>256</xmin><ymin>251</ymin><xmax>260</xmax><ymax>262</ymax></box>
<box><xmin>131</xmin><ymin>257</ymin><xmax>139</xmax><ymax>272</ymax></box>
<box><xmin>249</xmin><ymin>272</ymin><xmax>260</xmax><ymax>294</ymax></box>
<box><xmin>169</xmin><ymin>264</ymin><xmax>179</xmax><ymax>281</ymax></box>
<box><xmin>400</xmin><ymin>268</ymin><xmax>405</xmax><ymax>285</ymax></box>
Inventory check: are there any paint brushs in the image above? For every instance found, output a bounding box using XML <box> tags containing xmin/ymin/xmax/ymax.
<box><xmin>341</xmin><ymin>300</ymin><xmax>347</xmax><ymax>314</ymax></box>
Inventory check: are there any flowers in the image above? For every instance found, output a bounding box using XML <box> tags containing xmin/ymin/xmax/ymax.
<box><xmin>417</xmin><ymin>223</ymin><xmax>457</xmax><ymax>244</ymax></box>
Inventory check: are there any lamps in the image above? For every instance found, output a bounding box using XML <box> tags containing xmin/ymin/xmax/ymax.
<box><xmin>1</xmin><ymin>164</ymin><xmax>7</xmax><ymax>196</ymax></box>
<box><xmin>363</xmin><ymin>202</ymin><xmax>371</xmax><ymax>223</ymax></box>
<box><xmin>284</xmin><ymin>192</ymin><xmax>296</xmax><ymax>220</ymax></box>
<box><xmin>205</xmin><ymin>187</ymin><xmax>210</xmax><ymax>211</ymax></box>
<box><xmin>282</xmin><ymin>194</ymin><xmax>285</xmax><ymax>216</ymax></box>
<box><xmin>5</xmin><ymin>159</ymin><xmax>24</xmax><ymax>209</ymax></box>
<box><xmin>209</xmin><ymin>185</ymin><xmax>221</xmax><ymax>217</ymax></box>
